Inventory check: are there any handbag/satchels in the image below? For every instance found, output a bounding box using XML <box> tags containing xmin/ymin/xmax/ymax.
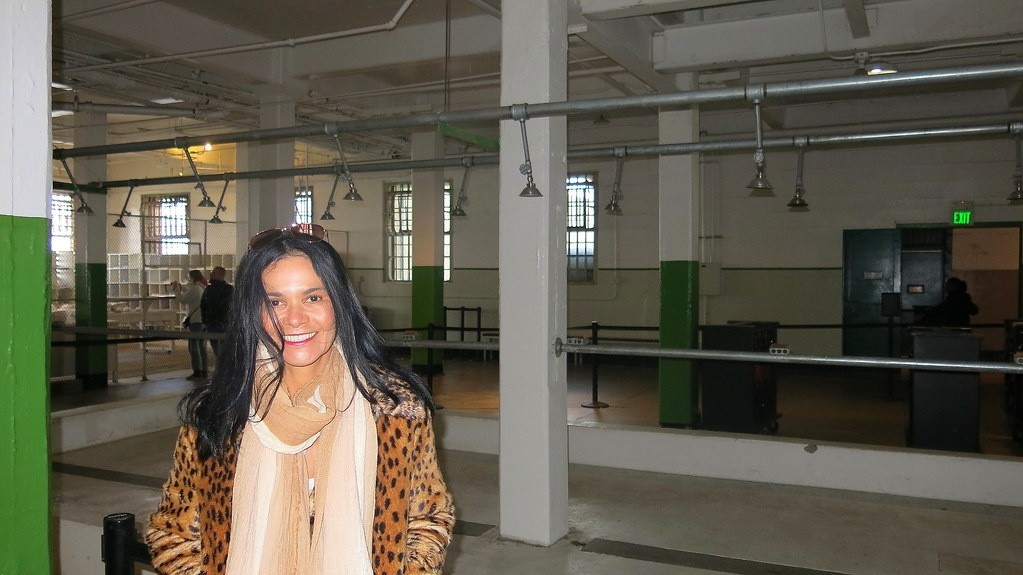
<box><xmin>182</xmin><ymin>316</ymin><xmax>190</xmax><ymax>328</ymax></box>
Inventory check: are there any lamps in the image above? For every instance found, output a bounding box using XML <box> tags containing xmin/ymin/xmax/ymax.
<box><xmin>450</xmin><ymin>165</ymin><xmax>472</xmax><ymax>216</ymax></box>
<box><xmin>745</xmin><ymin>103</ymin><xmax>773</xmax><ymax>189</ymax></box>
<box><xmin>182</xmin><ymin>146</ymin><xmax>216</xmax><ymax>207</ymax></box>
<box><xmin>867</xmin><ymin>60</ymin><xmax>897</xmax><ymax>76</ymax></box>
<box><xmin>60</xmin><ymin>159</ymin><xmax>92</xmax><ymax>213</ymax></box>
<box><xmin>951</xmin><ymin>200</ymin><xmax>976</xmax><ymax>226</ymax></box>
<box><xmin>1006</xmin><ymin>135</ymin><xmax>1023</xmax><ymax>200</ymax></box>
<box><xmin>605</xmin><ymin>157</ymin><xmax>625</xmax><ymax>212</ymax></box>
<box><xmin>333</xmin><ymin>134</ymin><xmax>363</xmax><ymax>202</ymax></box>
<box><xmin>209</xmin><ymin>179</ymin><xmax>229</xmax><ymax>224</ymax></box>
<box><xmin>112</xmin><ymin>186</ymin><xmax>134</xmax><ymax>229</ymax></box>
<box><xmin>518</xmin><ymin>118</ymin><xmax>543</xmax><ymax>198</ymax></box>
<box><xmin>320</xmin><ymin>174</ymin><xmax>341</xmax><ymax>220</ymax></box>
<box><xmin>786</xmin><ymin>147</ymin><xmax>808</xmax><ymax>207</ymax></box>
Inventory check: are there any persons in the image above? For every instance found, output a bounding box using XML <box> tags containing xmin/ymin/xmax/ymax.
<box><xmin>929</xmin><ymin>277</ymin><xmax>979</xmax><ymax>333</ymax></box>
<box><xmin>172</xmin><ymin>270</ymin><xmax>208</xmax><ymax>380</ymax></box>
<box><xmin>200</xmin><ymin>266</ymin><xmax>235</xmax><ymax>355</ymax></box>
<box><xmin>143</xmin><ymin>221</ymin><xmax>457</xmax><ymax>575</ymax></box>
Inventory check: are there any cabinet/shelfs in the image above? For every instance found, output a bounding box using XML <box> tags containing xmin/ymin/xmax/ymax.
<box><xmin>108</xmin><ymin>253</ymin><xmax>236</xmax><ymax>332</ymax></box>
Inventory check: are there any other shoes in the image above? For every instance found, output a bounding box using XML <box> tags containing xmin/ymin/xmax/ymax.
<box><xmin>186</xmin><ymin>370</ymin><xmax>202</xmax><ymax>380</ymax></box>
<box><xmin>201</xmin><ymin>371</ymin><xmax>208</xmax><ymax>378</ymax></box>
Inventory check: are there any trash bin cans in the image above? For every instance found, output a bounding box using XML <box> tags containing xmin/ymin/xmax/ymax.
<box><xmin>909</xmin><ymin>332</ymin><xmax>980</xmax><ymax>453</ymax></box>
<box><xmin>696</xmin><ymin>325</ymin><xmax>777</xmax><ymax>435</ymax></box>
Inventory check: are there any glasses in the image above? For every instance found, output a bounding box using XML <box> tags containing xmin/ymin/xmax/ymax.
<box><xmin>247</xmin><ymin>223</ymin><xmax>329</xmax><ymax>253</ymax></box>
